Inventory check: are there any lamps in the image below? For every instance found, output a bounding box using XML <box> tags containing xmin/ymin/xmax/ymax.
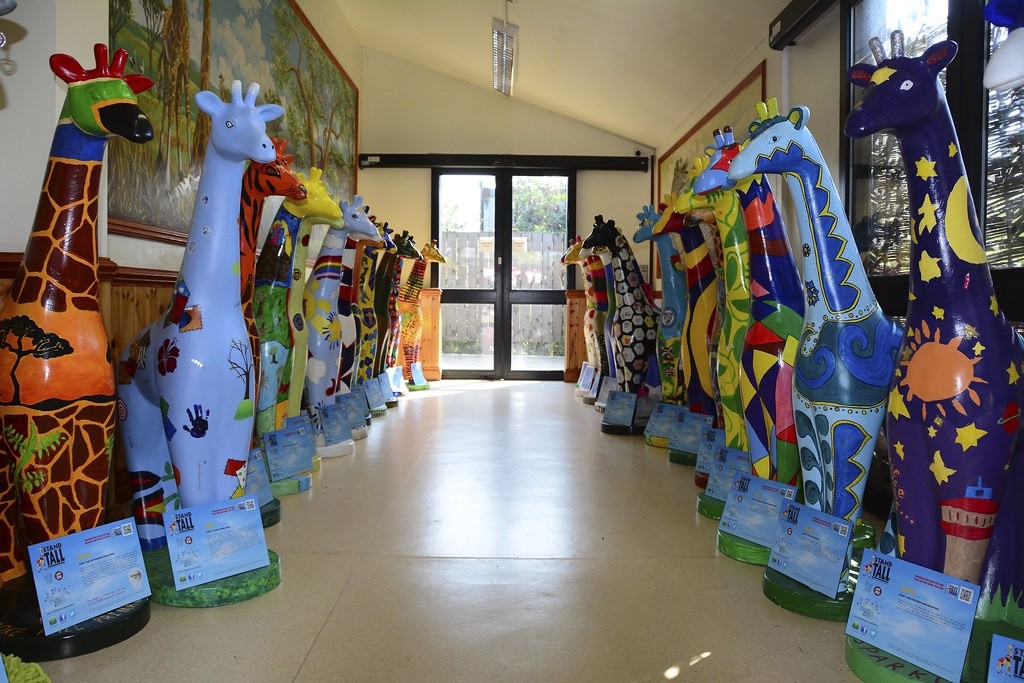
<box><xmin>492</xmin><ymin>17</ymin><xmax>519</xmax><ymax>97</ymax></box>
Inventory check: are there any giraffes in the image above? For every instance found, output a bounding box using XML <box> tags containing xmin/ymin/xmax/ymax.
<box><xmin>557</xmin><ymin>30</ymin><xmax>1023</xmax><ymax>681</ymax></box>
<box><xmin>1</xmin><ymin>41</ymin><xmax>449</xmax><ymax>663</ymax></box>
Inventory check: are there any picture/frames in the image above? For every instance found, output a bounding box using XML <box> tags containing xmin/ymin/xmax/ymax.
<box><xmin>108</xmin><ymin>0</ymin><xmax>359</xmax><ymax>247</ymax></box>
<box><xmin>656</xmin><ymin>59</ymin><xmax>767</xmax><ymax>279</ymax></box>
